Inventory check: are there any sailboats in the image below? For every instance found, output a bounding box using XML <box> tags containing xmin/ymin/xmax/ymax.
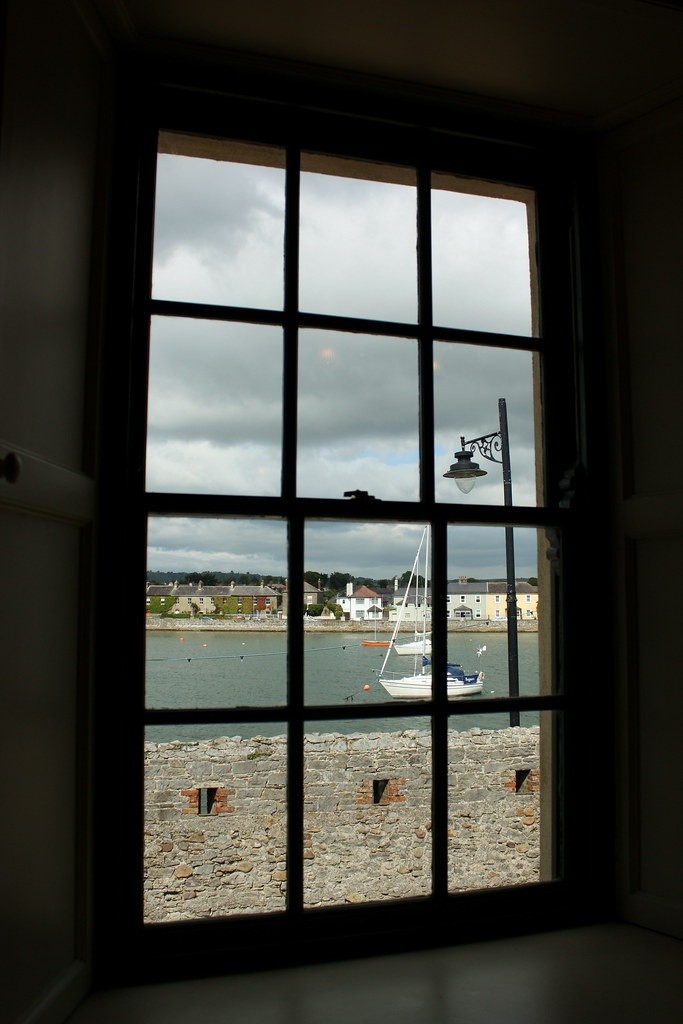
<box><xmin>392</xmin><ymin>553</ymin><xmax>432</xmax><ymax>655</ymax></box>
<box><xmin>361</xmin><ymin>598</ymin><xmax>392</xmax><ymax>648</ymax></box>
<box><xmin>379</xmin><ymin>524</ymin><xmax>483</xmax><ymax>700</ymax></box>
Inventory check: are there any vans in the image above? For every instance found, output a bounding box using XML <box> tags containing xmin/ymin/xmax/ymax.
<box><xmin>495</xmin><ymin>617</ymin><xmax>508</xmax><ymax>621</ymax></box>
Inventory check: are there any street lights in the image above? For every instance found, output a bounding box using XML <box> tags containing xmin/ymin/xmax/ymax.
<box><xmin>443</xmin><ymin>431</ymin><xmax>519</xmax><ymax>729</ymax></box>
